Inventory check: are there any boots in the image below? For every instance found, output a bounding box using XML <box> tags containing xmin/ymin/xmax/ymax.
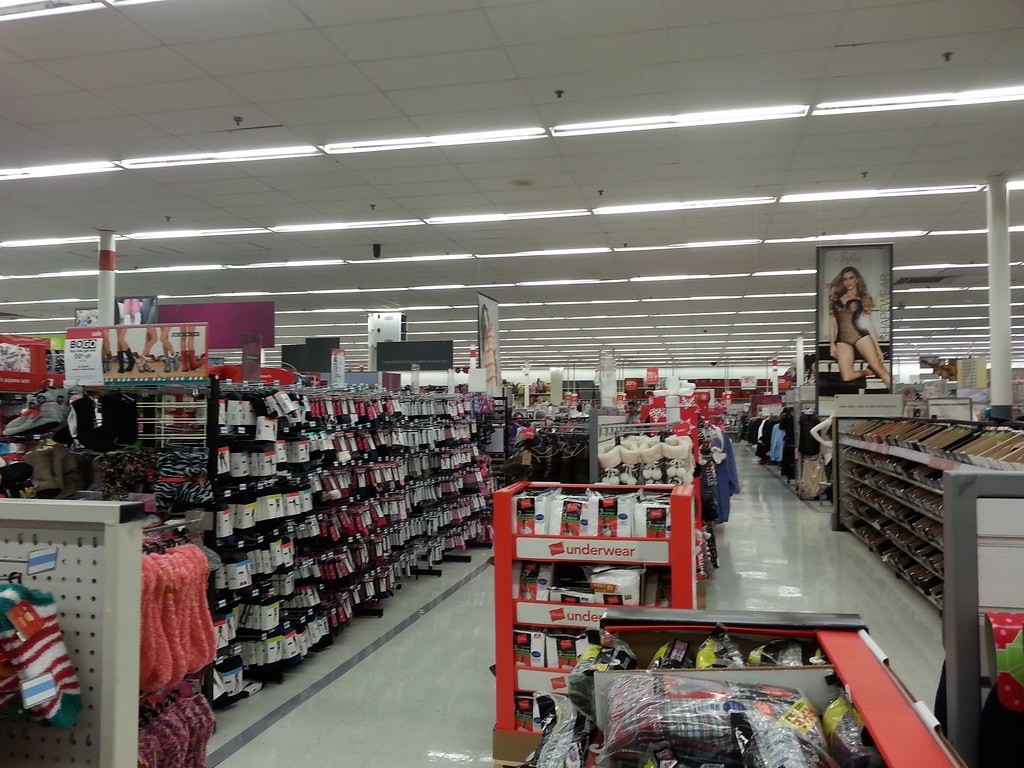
<box><xmin>136</xmin><ymin>354</ymin><xmax>145</xmax><ymax>373</ymax></box>
<box><xmin>124</xmin><ymin>348</ymin><xmax>135</xmax><ymax>372</ymax></box>
<box><xmin>144</xmin><ymin>354</ymin><xmax>155</xmax><ymax>372</ymax></box>
<box><xmin>102</xmin><ymin>357</ymin><xmax>107</xmax><ymax>373</ymax></box>
<box><xmin>107</xmin><ymin>351</ymin><xmax>112</xmax><ymax>371</ymax></box>
<box><xmin>162</xmin><ymin>358</ymin><xmax>172</xmax><ymax>372</ymax></box>
<box><xmin>188</xmin><ymin>350</ymin><xmax>204</xmax><ymax>370</ymax></box>
<box><xmin>180</xmin><ymin>350</ymin><xmax>189</xmax><ymax>371</ymax></box>
<box><xmin>117</xmin><ymin>351</ymin><xmax>124</xmax><ymax>373</ymax></box>
<box><xmin>169</xmin><ymin>352</ymin><xmax>179</xmax><ymax>370</ymax></box>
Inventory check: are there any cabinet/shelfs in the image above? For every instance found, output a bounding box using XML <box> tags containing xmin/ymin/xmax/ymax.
<box><xmin>0</xmin><ymin>378</ymin><xmax>1022</xmax><ymax>768</ymax></box>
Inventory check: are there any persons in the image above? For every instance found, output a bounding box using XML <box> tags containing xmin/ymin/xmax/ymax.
<box><xmin>513</xmin><ymin>411</ymin><xmax>554</xmax><ymax>426</ymax></box>
<box><xmin>480</xmin><ymin>304</ymin><xmax>497</xmax><ymax>392</ymax></box>
<box><xmin>810</xmin><ymin>415</ymin><xmax>833</xmax><ymax>479</ymax></box>
<box><xmin>828</xmin><ymin>266</ymin><xmax>890</xmax><ymax>386</ymax></box>
<box><xmin>101</xmin><ymin>326</ymin><xmax>205</xmax><ymax>372</ymax></box>
<box><xmin>800</xmin><ymin>411</ymin><xmax>821</xmax><ymax>455</ymax></box>
<box><xmin>624</xmin><ymin>402</ymin><xmax>638</xmax><ymax>423</ymax></box>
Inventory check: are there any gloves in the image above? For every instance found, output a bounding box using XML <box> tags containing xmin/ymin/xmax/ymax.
<box><xmin>598</xmin><ymin>433</ymin><xmax>695</xmax><ymax>486</ymax></box>
